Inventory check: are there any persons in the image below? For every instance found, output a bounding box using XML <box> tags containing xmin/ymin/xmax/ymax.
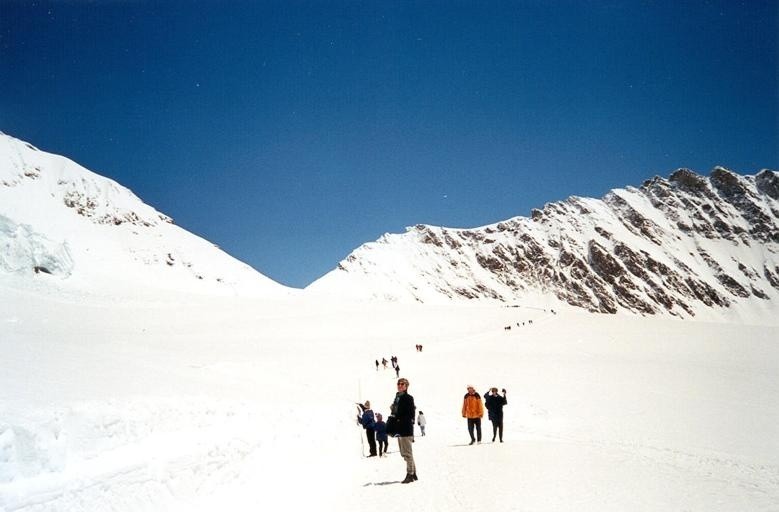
<box><xmin>354</xmin><ymin>345</ymin><xmax>508</xmax><ymax>484</ymax></box>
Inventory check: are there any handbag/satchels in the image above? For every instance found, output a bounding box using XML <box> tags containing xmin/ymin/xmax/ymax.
<box><xmin>384</xmin><ymin>415</ymin><xmax>398</xmax><ymax>438</ymax></box>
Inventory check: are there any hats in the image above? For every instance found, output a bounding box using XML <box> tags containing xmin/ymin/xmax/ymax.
<box><xmin>374</xmin><ymin>412</ymin><xmax>383</xmax><ymax>420</ymax></box>
<box><xmin>363</xmin><ymin>400</ymin><xmax>371</xmax><ymax>408</ymax></box>
